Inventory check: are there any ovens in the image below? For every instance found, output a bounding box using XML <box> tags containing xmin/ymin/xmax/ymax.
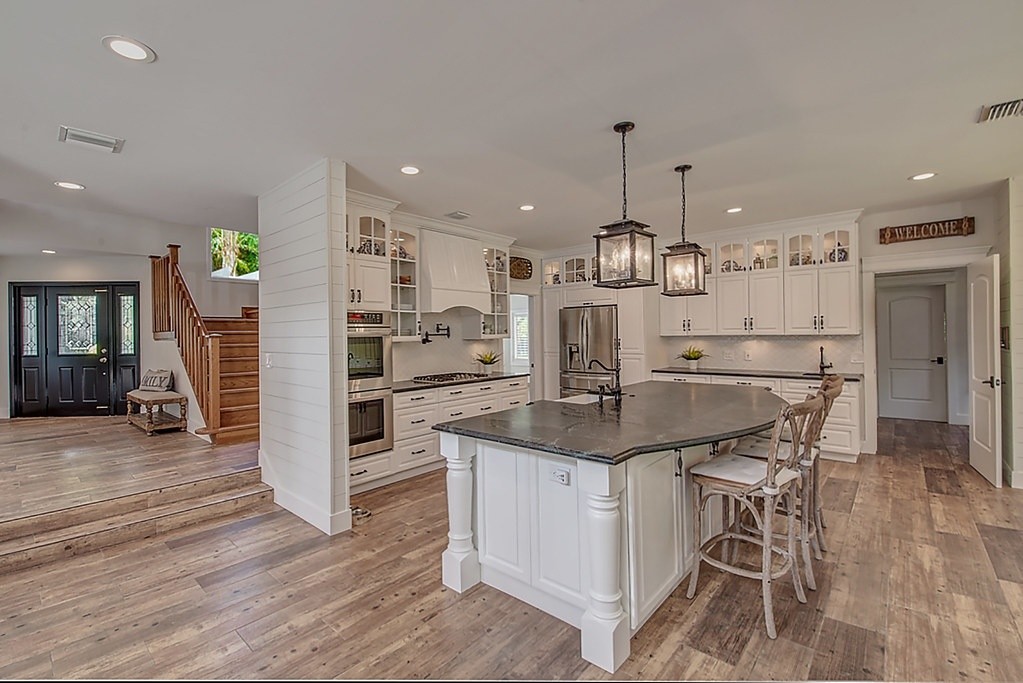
<box><xmin>346</xmin><ymin>311</ymin><xmax>395</xmax><ymax>459</ymax></box>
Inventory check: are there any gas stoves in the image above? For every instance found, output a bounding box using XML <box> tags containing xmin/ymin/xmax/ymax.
<box><xmin>413</xmin><ymin>371</ymin><xmax>489</xmax><ymax>385</ymax></box>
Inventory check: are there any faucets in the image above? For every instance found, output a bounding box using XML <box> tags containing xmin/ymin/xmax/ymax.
<box><xmin>588</xmin><ymin>358</ymin><xmax>622</xmax><ymax>401</ymax></box>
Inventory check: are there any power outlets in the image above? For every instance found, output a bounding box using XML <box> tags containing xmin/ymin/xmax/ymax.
<box><xmin>472</xmin><ymin>354</ymin><xmax>476</xmax><ymax>364</ymax></box>
<box><xmin>744</xmin><ymin>350</ymin><xmax>752</xmax><ymax>361</ymax></box>
<box><xmin>723</xmin><ymin>351</ymin><xmax>734</xmax><ymax>360</ymax></box>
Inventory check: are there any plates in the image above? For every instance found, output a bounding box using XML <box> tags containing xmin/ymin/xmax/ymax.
<box><xmin>391</xmin><ymin>327</ymin><xmax>411</xmax><ymax>336</ymax></box>
<box><xmin>722</xmin><ymin>261</ymin><xmax>738</xmax><ymax>272</ymax></box>
<box><xmin>392</xmin><ymin>303</ymin><xmax>413</xmax><ymax>311</ymax></box>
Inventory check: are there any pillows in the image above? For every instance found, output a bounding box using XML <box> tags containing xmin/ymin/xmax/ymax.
<box><xmin>139</xmin><ymin>368</ymin><xmax>175</xmax><ymax>392</ymax></box>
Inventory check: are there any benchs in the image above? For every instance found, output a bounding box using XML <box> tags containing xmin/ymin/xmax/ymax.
<box><xmin>126</xmin><ymin>388</ymin><xmax>188</xmax><ymax>438</ymax></box>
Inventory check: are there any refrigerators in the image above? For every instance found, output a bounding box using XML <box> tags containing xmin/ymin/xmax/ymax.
<box><xmin>558</xmin><ymin>305</ymin><xmax>620</xmax><ymax>399</ymax></box>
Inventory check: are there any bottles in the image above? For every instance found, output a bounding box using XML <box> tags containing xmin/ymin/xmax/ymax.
<box><xmin>830</xmin><ymin>242</ymin><xmax>847</xmax><ymax>263</ymax></box>
<box><xmin>754</xmin><ymin>253</ymin><xmax>765</xmax><ymax>269</ymax></box>
<box><xmin>492</xmin><ymin>256</ymin><xmax>503</xmax><ymax>271</ymax></box>
<box><xmin>363</xmin><ymin>234</ymin><xmax>379</xmax><ymax>255</ymax></box>
<box><xmin>491</xmin><ymin>279</ymin><xmax>495</xmax><ymax>291</ymax></box>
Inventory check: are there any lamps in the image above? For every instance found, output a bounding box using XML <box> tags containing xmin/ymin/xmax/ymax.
<box><xmin>662</xmin><ymin>163</ymin><xmax>708</xmax><ymax>297</ymax></box>
<box><xmin>593</xmin><ymin>121</ymin><xmax>659</xmax><ymax>290</ymax></box>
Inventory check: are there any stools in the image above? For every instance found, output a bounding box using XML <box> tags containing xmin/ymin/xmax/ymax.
<box><xmin>688</xmin><ymin>375</ymin><xmax>845</xmax><ymax>641</ymax></box>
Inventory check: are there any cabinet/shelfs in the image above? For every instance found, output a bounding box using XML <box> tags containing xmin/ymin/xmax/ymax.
<box><xmin>438</xmin><ymin>379</ymin><xmax>791</xmax><ymax>675</ymax></box>
<box><xmin>540</xmin><ymin>282</ymin><xmax>645</xmax><ymax>401</ymax></box>
<box><xmin>660</xmin><ymin>206</ymin><xmax>865</xmax><ymax>465</ymax></box>
<box><xmin>344</xmin><ymin>186</ymin><xmax>516</xmax><ymax>343</ymax></box>
<box><xmin>350</xmin><ymin>376</ymin><xmax>530</xmax><ymax>497</ymax></box>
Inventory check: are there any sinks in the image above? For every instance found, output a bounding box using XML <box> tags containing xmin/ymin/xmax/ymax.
<box><xmin>553</xmin><ymin>394</ymin><xmax>615</xmax><ymax>405</ymax></box>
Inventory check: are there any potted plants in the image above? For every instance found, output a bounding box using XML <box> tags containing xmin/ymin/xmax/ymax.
<box><xmin>674</xmin><ymin>345</ymin><xmax>711</xmax><ymax>369</ymax></box>
<box><xmin>475</xmin><ymin>350</ymin><xmax>504</xmax><ymax>375</ymax></box>
